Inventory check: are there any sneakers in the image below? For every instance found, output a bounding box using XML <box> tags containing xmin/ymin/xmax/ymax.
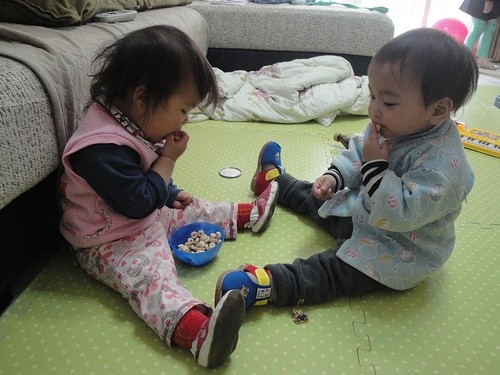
<box><xmin>244</xmin><ymin>181</ymin><xmax>278</xmax><ymax>234</ymax></box>
<box><xmin>251</xmin><ymin>140</ymin><xmax>286</xmax><ymax>195</ymax></box>
<box><xmin>185</xmin><ymin>288</ymin><xmax>245</xmax><ymax>367</ymax></box>
<box><xmin>215</xmin><ymin>263</ymin><xmax>272</xmax><ymax>309</ymax></box>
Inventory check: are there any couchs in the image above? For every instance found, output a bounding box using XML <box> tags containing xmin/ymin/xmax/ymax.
<box><xmin>0</xmin><ymin>0</ymin><xmax>395</xmax><ymax>210</ymax></box>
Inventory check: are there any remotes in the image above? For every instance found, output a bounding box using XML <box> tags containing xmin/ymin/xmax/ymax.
<box><xmin>95</xmin><ymin>9</ymin><xmax>138</xmax><ymax>23</ymax></box>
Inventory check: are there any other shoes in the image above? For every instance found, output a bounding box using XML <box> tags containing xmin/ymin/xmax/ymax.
<box><xmin>476</xmin><ymin>59</ymin><xmax>499</xmax><ymax>70</ymax></box>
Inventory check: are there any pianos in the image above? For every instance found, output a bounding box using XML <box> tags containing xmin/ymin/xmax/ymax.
<box><xmin>455</xmin><ymin>120</ymin><xmax>500</xmax><ymax>159</ymax></box>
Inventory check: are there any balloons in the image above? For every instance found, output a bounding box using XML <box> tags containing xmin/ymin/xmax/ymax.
<box><xmin>433</xmin><ymin>18</ymin><xmax>468</xmax><ymax>43</ymax></box>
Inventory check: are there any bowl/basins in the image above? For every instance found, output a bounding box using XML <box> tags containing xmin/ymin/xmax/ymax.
<box><xmin>168</xmin><ymin>222</ymin><xmax>226</xmax><ymax>266</ymax></box>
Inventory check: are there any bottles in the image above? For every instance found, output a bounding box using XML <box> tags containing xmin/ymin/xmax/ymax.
<box><xmin>332</xmin><ymin>130</ymin><xmax>364</xmax><ymax>150</ymax></box>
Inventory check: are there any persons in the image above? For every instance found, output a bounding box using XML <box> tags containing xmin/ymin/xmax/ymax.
<box><xmin>458</xmin><ymin>0</ymin><xmax>500</xmax><ymax>70</ymax></box>
<box><xmin>215</xmin><ymin>28</ymin><xmax>479</xmax><ymax>311</ymax></box>
<box><xmin>59</xmin><ymin>25</ymin><xmax>279</xmax><ymax>368</ymax></box>
